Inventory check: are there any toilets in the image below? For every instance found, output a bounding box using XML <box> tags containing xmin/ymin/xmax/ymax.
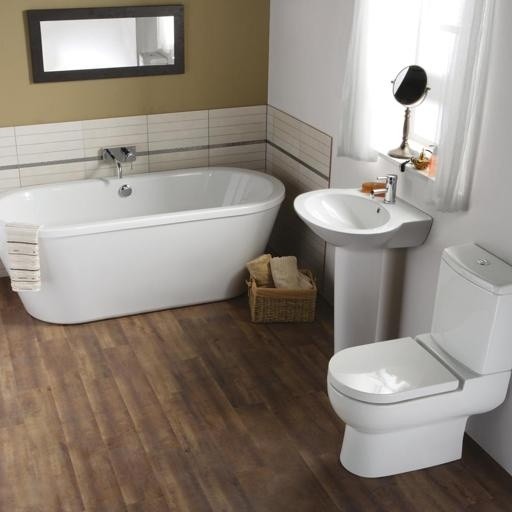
<box><xmin>326</xmin><ymin>243</ymin><xmax>512</xmax><ymax>478</ymax></box>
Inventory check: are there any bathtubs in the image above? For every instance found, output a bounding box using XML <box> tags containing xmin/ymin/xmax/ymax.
<box><xmin>0</xmin><ymin>167</ymin><xmax>285</xmax><ymax>324</ymax></box>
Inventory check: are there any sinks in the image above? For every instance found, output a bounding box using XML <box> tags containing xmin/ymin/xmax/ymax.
<box><xmin>294</xmin><ymin>188</ymin><xmax>432</xmax><ymax>249</ymax></box>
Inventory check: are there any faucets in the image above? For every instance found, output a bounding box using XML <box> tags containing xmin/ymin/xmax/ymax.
<box><xmin>104</xmin><ymin>149</ymin><xmax>123</xmax><ymax>179</ymax></box>
<box><xmin>371</xmin><ymin>174</ymin><xmax>398</xmax><ymax>203</ymax></box>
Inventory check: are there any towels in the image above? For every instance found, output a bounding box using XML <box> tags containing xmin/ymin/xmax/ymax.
<box><xmin>247</xmin><ymin>252</ymin><xmax>314</xmax><ymax>296</ymax></box>
<box><xmin>4</xmin><ymin>220</ymin><xmax>42</xmax><ymax>293</ymax></box>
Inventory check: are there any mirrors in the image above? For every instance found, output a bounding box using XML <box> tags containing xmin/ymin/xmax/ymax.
<box><xmin>25</xmin><ymin>4</ymin><xmax>185</xmax><ymax>83</ymax></box>
<box><xmin>385</xmin><ymin>64</ymin><xmax>429</xmax><ymax>160</ymax></box>
